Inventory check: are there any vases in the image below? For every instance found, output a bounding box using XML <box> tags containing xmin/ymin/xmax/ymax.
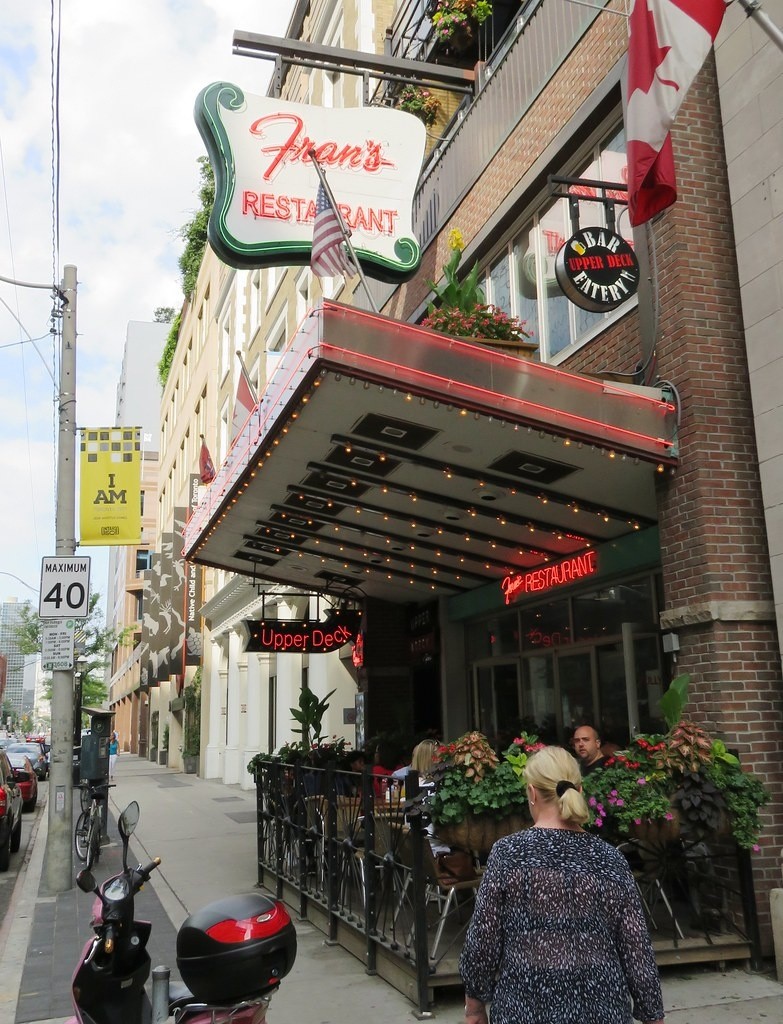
<box><xmin>448</xmin><ymin>27</ymin><xmax>475</xmax><ymax>49</ymax></box>
<box><xmin>632</xmin><ymin>790</ymin><xmax>680</xmax><ymax>840</ymax></box>
<box><xmin>449</xmin><ymin>814</ymin><xmax>533</xmax><ymax>850</ymax></box>
<box><xmin>462</xmin><ymin>337</ymin><xmax>540</xmax><ymax>358</ymax></box>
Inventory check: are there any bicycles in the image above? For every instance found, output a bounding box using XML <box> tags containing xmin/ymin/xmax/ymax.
<box><xmin>73</xmin><ymin>784</ymin><xmax>118</xmax><ymax>869</ymax></box>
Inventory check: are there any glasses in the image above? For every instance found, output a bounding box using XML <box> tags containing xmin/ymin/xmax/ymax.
<box><xmin>434</xmin><ymin>739</ymin><xmax>441</xmax><ymax>752</ymax></box>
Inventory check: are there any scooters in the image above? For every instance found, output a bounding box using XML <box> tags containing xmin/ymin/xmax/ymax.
<box><xmin>64</xmin><ymin>800</ymin><xmax>296</xmax><ymax>1024</ymax></box>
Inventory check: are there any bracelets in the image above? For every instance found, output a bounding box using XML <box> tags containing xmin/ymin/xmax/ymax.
<box><xmin>465</xmin><ymin>1003</ymin><xmax>484</xmax><ymax>1017</ymax></box>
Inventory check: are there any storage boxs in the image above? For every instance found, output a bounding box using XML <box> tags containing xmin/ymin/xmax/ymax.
<box><xmin>177</xmin><ymin>890</ymin><xmax>297</xmax><ymax>1004</ymax></box>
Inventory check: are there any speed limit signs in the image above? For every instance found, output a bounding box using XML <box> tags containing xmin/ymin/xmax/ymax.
<box><xmin>38</xmin><ymin>556</ymin><xmax>92</xmax><ymax>620</ymax></box>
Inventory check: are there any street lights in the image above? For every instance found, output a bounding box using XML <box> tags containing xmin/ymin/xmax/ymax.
<box><xmin>74</xmin><ymin>655</ymin><xmax>88</xmax><ymax>761</ymax></box>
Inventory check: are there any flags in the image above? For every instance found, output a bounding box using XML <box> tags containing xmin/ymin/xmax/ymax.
<box><xmin>628</xmin><ymin>0</ymin><xmax>726</xmax><ymax>228</ymax></box>
<box><xmin>232</xmin><ymin>367</ymin><xmax>258</xmax><ymax>432</ymax></box>
<box><xmin>310</xmin><ymin>182</ymin><xmax>357</xmax><ymax>278</ymax></box>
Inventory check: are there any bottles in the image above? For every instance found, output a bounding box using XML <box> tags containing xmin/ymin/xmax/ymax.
<box><xmin>385</xmin><ymin>787</ymin><xmax>390</xmax><ymax>803</ymax></box>
<box><xmin>392</xmin><ymin>787</ymin><xmax>399</xmax><ymax>803</ymax></box>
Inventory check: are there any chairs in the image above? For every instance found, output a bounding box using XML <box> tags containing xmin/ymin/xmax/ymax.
<box><xmin>282</xmin><ymin>795</ymin><xmax>485</xmax><ymax>960</ymax></box>
<box><xmin>614</xmin><ymin>838</ymin><xmax>683</xmax><ymax>941</ymax></box>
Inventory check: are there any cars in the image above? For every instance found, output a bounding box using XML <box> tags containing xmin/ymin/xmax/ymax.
<box><xmin>6</xmin><ymin>753</ymin><xmax>38</xmax><ymax>812</ymax></box>
<box><xmin>0</xmin><ymin>747</ymin><xmax>22</xmax><ymax>874</ymax></box>
<box><xmin>74</xmin><ymin>729</ymin><xmax>91</xmax><ymax>735</ymax></box>
<box><xmin>0</xmin><ymin>736</ymin><xmax>51</xmax><ymax>781</ymax></box>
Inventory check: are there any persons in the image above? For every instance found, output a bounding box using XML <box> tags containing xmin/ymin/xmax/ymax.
<box><xmin>458</xmin><ymin>744</ymin><xmax>665</xmax><ymax>1024</ymax></box>
<box><xmin>346</xmin><ymin>724</ymin><xmax>620</xmax><ymax>918</ymax></box>
<box><xmin>108</xmin><ymin>729</ymin><xmax>121</xmax><ymax>782</ymax></box>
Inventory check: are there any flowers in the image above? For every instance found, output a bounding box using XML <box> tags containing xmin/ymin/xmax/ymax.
<box><xmin>421</xmin><ymin>231</ymin><xmax>534</xmax><ymax>341</ymax></box>
<box><xmin>403</xmin><ymin>731</ymin><xmax>545</xmax><ymax>826</ymax></box>
<box><xmin>581</xmin><ymin>673</ymin><xmax>772</xmax><ymax>855</ymax></box>
<box><xmin>430</xmin><ymin>0</ymin><xmax>494</xmax><ymax>41</ymax></box>
<box><xmin>398</xmin><ymin>85</ymin><xmax>439</xmax><ymax>127</ymax></box>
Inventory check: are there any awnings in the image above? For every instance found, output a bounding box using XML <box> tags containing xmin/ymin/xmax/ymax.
<box><xmin>198</xmin><ymin>435</ymin><xmax>215</xmax><ymax>484</ymax></box>
